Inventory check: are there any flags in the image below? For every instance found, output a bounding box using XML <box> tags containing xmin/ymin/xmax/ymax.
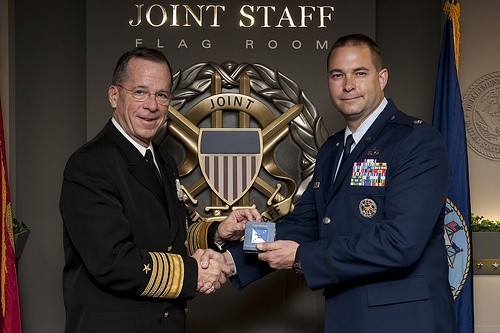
<box><xmin>0</xmin><ymin>111</ymin><xmax>21</xmax><ymax>333</ymax></box>
<box><xmin>433</xmin><ymin>0</ymin><xmax>474</xmax><ymax>333</ymax></box>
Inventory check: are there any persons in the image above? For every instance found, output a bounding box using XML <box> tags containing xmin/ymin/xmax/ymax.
<box><xmin>197</xmin><ymin>34</ymin><xmax>457</xmax><ymax>333</ymax></box>
<box><xmin>60</xmin><ymin>46</ymin><xmax>261</xmax><ymax>333</ymax></box>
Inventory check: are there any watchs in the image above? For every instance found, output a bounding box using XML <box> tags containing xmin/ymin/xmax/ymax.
<box><xmin>292</xmin><ymin>246</ymin><xmax>302</xmax><ymax>272</ymax></box>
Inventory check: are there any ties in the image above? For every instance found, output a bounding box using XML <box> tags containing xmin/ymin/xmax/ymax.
<box><xmin>144</xmin><ymin>149</ymin><xmax>160</xmax><ymax>185</ymax></box>
<box><xmin>338</xmin><ymin>133</ymin><xmax>355</xmax><ymax>171</ymax></box>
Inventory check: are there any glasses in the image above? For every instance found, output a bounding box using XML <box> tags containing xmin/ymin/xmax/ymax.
<box><xmin>114</xmin><ymin>83</ymin><xmax>173</xmax><ymax>106</ymax></box>
